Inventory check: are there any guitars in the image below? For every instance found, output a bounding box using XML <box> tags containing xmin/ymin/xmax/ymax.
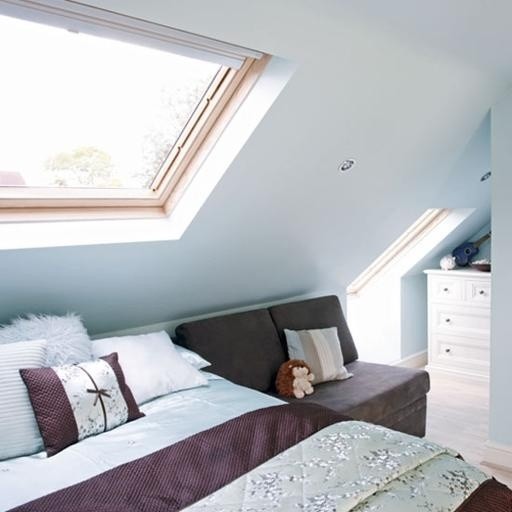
<box><xmin>452</xmin><ymin>231</ymin><xmax>491</xmax><ymax>266</ymax></box>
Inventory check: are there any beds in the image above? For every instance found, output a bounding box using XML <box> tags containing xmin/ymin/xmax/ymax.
<box><xmin>1</xmin><ymin>328</ymin><xmax>511</xmax><ymax>512</ymax></box>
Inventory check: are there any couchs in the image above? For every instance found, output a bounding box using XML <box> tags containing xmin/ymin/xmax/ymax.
<box><xmin>175</xmin><ymin>295</ymin><xmax>430</xmax><ymax>439</ymax></box>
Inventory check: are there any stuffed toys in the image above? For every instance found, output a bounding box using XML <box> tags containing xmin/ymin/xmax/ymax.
<box><xmin>272</xmin><ymin>358</ymin><xmax>317</xmax><ymax>400</ymax></box>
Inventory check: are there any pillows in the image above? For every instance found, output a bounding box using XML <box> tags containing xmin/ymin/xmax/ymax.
<box><xmin>2</xmin><ymin>308</ymin><xmax>211</xmax><ymax>459</ymax></box>
<box><xmin>284</xmin><ymin>326</ymin><xmax>355</xmax><ymax>384</ymax></box>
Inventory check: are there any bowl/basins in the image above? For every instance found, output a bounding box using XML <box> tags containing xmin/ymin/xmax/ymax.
<box><xmin>469</xmin><ymin>263</ymin><xmax>490</xmax><ymax>272</ymax></box>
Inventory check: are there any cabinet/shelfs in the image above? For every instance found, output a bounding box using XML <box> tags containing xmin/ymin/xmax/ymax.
<box><xmin>422</xmin><ymin>268</ymin><xmax>491</xmax><ymax>379</ymax></box>
